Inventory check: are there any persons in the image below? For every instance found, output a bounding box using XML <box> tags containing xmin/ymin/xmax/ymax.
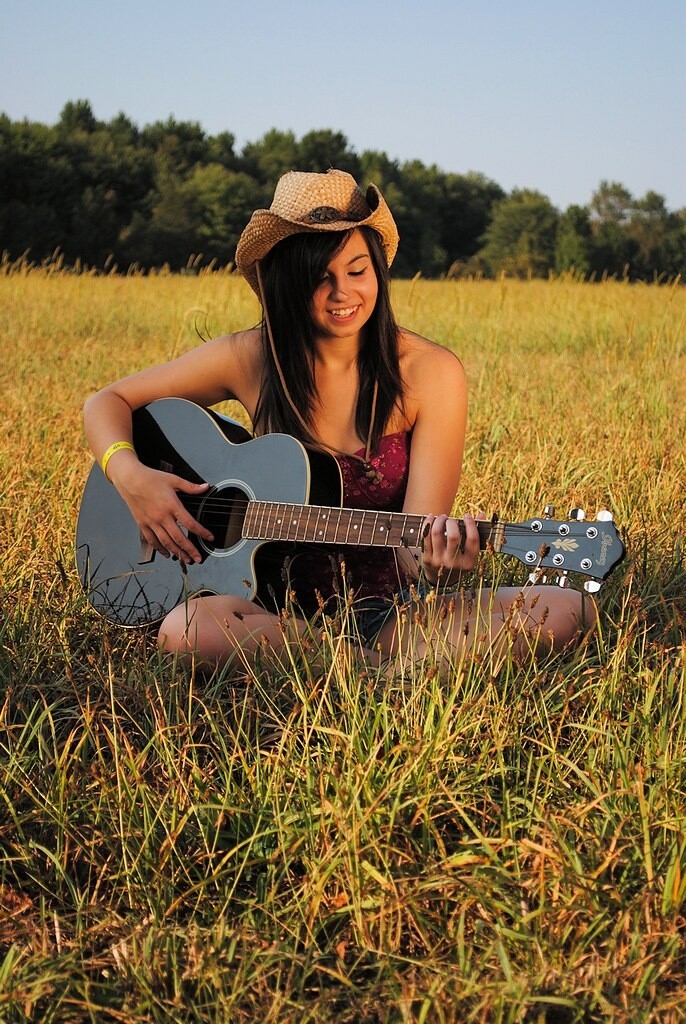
<box><xmin>83</xmin><ymin>170</ymin><xmax>597</xmax><ymax>689</ymax></box>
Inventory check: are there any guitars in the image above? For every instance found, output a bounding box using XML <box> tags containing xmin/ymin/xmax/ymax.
<box><xmin>74</xmin><ymin>396</ymin><xmax>627</xmax><ymax>632</ymax></box>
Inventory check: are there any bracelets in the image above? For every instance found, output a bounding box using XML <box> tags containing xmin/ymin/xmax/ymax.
<box><xmin>101</xmin><ymin>441</ymin><xmax>135</xmax><ymax>483</ymax></box>
<box><xmin>422</xmin><ymin>567</ymin><xmax>460</xmax><ymax>588</ymax></box>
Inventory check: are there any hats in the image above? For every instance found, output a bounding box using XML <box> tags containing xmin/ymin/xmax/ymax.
<box><xmin>234</xmin><ymin>170</ymin><xmax>400</xmax><ymax>478</ymax></box>
